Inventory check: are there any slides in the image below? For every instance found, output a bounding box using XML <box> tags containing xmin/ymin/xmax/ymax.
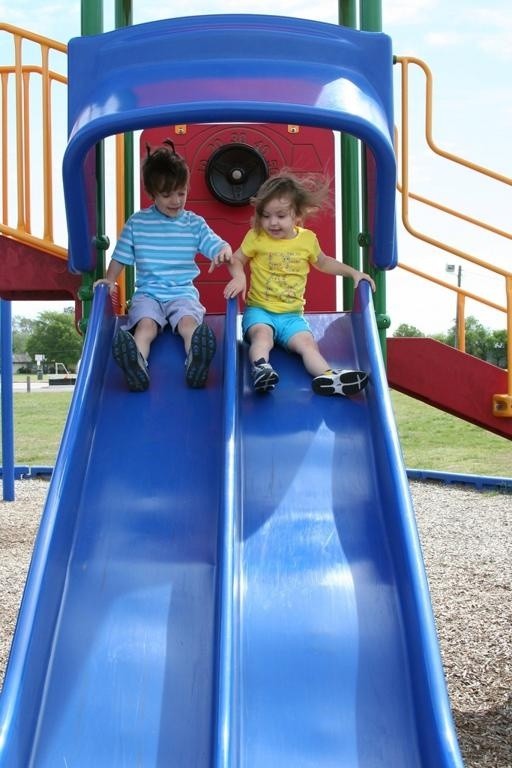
<box><xmin>0</xmin><ymin>272</ymin><xmax>464</xmax><ymax>767</ymax></box>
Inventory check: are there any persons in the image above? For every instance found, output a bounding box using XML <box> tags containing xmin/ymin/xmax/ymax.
<box><xmin>224</xmin><ymin>166</ymin><xmax>377</xmax><ymax>397</ymax></box>
<box><xmin>91</xmin><ymin>137</ymin><xmax>232</xmax><ymax>393</ymax></box>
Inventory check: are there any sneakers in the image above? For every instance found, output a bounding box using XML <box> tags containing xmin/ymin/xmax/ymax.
<box><xmin>311</xmin><ymin>368</ymin><xmax>369</xmax><ymax>397</ymax></box>
<box><xmin>251</xmin><ymin>363</ymin><xmax>279</xmax><ymax>393</ymax></box>
<box><xmin>112</xmin><ymin>329</ymin><xmax>151</xmax><ymax>392</ymax></box>
<box><xmin>185</xmin><ymin>323</ymin><xmax>217</xmax><ymax>389</ymax></box>
